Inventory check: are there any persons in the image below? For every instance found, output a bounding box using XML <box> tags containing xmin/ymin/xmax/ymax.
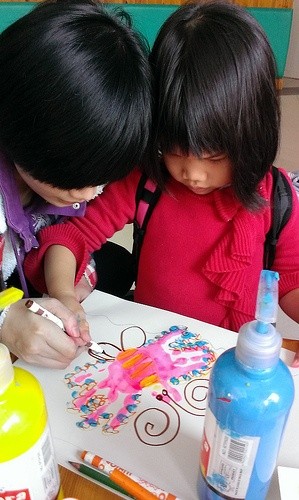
<box><xmin>0</xmin><ymin>0</ymin><xmax>155</xmax><ymax>370</ymax></box>
<box><xmin>24</xmin><ymin>2</ymin><xmax>299</xmax><ymax>368</ymax></box>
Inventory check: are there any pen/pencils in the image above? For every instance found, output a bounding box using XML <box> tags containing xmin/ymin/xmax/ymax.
<box><xmin>68</xmin><ymin>451</ymin><xmax>180</xmax><ymax>500</ymax></box>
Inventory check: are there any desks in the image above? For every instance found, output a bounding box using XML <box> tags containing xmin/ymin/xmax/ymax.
<box><xmin>10</xmin><ymin>339</ymin><xmax>299</xmax><ymax>500</ymax></box>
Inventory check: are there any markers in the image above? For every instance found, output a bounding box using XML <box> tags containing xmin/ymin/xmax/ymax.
<box><xmin>24</xmin><ymin>300</ymin><xmax>104</xmax><ymax>354</ymax></box>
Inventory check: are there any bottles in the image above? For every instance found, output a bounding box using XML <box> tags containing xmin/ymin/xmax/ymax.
<box><xmin>195</xmin><ymin>270</ymin><xmax>295</xmax><ymax>500</ymax></box>
